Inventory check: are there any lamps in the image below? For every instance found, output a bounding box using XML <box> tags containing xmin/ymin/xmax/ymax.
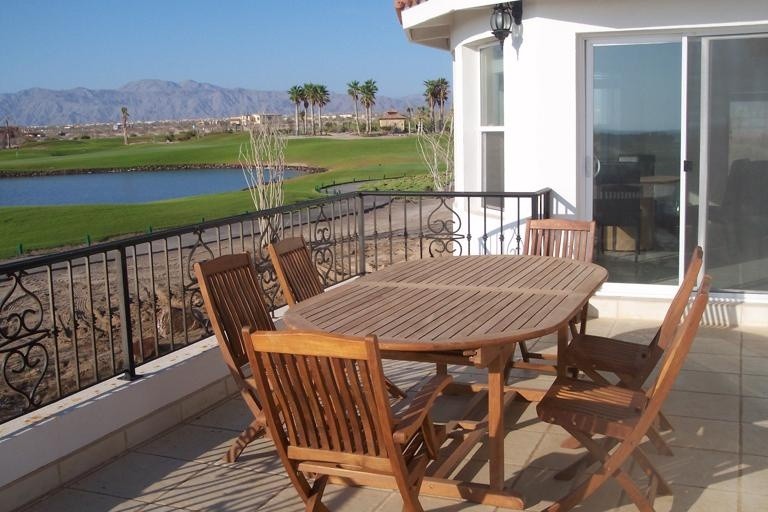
<box><xmin>489</xmin><ymin>0</ymin><xmax>522</xmax><ymax>50</ymax></box>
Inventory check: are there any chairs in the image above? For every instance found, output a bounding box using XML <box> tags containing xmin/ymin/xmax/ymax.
<box><xmin>593</xmin><ymin>163</ymin><xmax>641</xmax><ymax>262</ymax></box>
<box><xmin>617</xmin><ymin>153</ymin><xmax>655</xmax><ymax>177</ymax></box>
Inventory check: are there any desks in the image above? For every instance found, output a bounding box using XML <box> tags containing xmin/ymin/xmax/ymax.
<box><xmin>593</xmin><ymin>175</ymin><xmax>679</xmax><ymax>252</ymax></box>
